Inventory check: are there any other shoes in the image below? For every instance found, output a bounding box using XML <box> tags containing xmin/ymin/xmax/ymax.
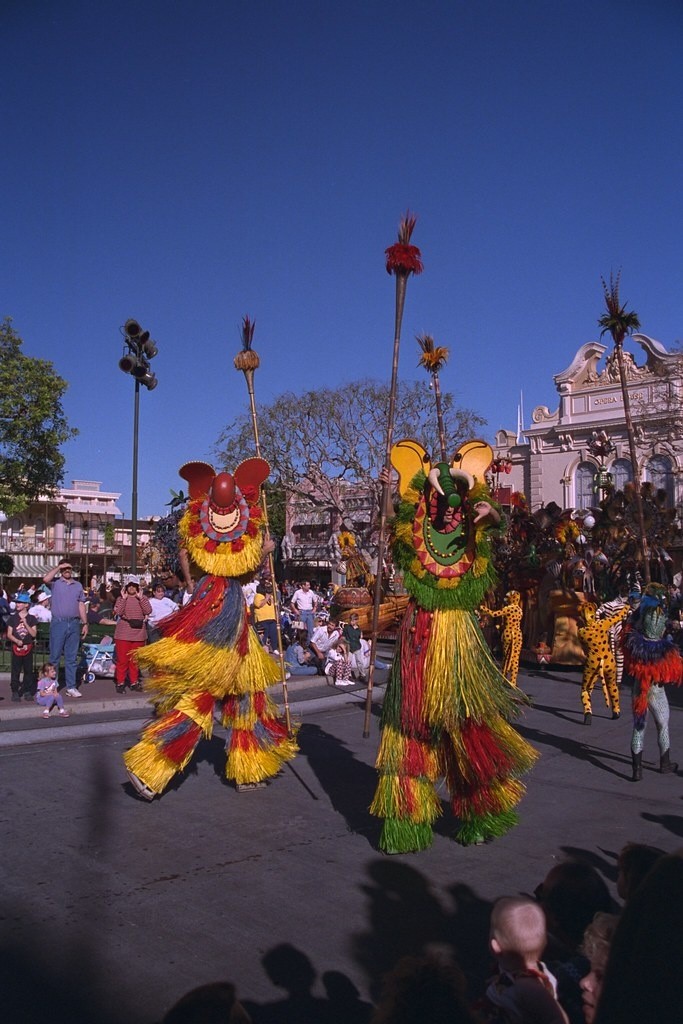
<box><xmin>612</xmin><ymin>711</ymin><xmax>622</xmax><ymax>720</ymax></box>
<box><xmin>273</xmin><ymin>649</ymin><xmax>280</xmax><ymax>655</ymax></box>
<box><xmin>345</xmin><ymin>679</ymin><xmax>356</xmax><ymax>685</ymax></box>
<box><xmin>130</xmin><ymin>682</ymin><xmax>144</xmax><ymax>692</ymax></box>
<box><xmin>23</xmin><ymin>691</ymin><xmax>35</xmax><ymax>702</ymax></box>
<box><xmin>584</xmin><ymin>712</ymin><xmax>593</xmax><ymax>726</ymax></box>
<box><xmin>42</xmin><ymin>711</ymin><xmax>54</xmax><ymax>719</ymax></box>
<box><xmin>59</xmin><ymin>711</ymin><xmax>69</xmax><ymax>718</ymax></box>
<box><xmin>10</xmin><ymin>693</ymin><xmax>21</xmax><ymax>702</ymax></box>
<box><xmin>66</xmin><ymin>688</ymin><xmax>83</xmax><ymax>698</ymax></box>
<box><xmin>118</xmin><ymin>685</ymin><xmax>127</xmax><ymax>694</ymax></box>
<box><xmin>385</xmin><ymin>663</ymin><xmax>393</xmax><ymax>670</ymax></box>
<box><xmin>335</xmin><ymin>679</ymin><xmax>350</xmax><ymax>687</ymax></box>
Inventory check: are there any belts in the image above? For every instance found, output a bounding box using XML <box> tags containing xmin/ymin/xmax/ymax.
<box><xmin>299</xmin><ymin>610</ymin><xmax>311</xmax><ymax>613</ymax></box>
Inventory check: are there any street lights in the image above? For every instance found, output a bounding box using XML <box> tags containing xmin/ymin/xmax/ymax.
<box><xmin>117</xmin><ymin>317</ymin><xmax>160</xmax><ymax>576</ymax></box>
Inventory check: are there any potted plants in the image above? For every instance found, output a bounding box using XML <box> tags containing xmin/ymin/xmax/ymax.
<box><xmin>103</xmin><ymin>523</ymin><xmax>114</xmax><ymax>550</ymax></box>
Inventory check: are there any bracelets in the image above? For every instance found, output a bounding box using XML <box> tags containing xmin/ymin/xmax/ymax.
<box><xmin>82</xmin><ymin>622</ymin><xmax>88</xmax><ymax>625</ymax></box>
<box><xmin>344</xmin><ymin>652</ymin><xmax>347</xmax><ymax>654</ymax></box>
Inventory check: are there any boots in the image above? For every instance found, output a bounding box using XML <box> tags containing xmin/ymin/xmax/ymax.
<box><xmin>630</xmin><ymin>748</ymin><xmax>646</xmax><ymax>780</ymax></box>
<box><xmin>659</xmin><ymin>747</ymin><xmax>679</xmax><ymax>773</ymax></box>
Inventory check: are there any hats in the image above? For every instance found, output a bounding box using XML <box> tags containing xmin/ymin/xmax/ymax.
<box><xmin>123</xmin><ymin>573</ymin><xmax>142</xmax><ymax>586</ymax></box>
<box><xmin>36</xmin><ymin>592</ymin><xmax>51</xmax><ymax>602</ymax></box>
<box><xmin>11</xmin><ymin>594</ymin><xmax>32</xmax><ymax>604</ymax></box>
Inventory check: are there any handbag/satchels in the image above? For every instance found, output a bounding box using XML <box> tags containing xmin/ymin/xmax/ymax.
<box><xmin>128</xmin><ymin>618</ymin><xmax>146</xmax><ymax>629</ymax></box>
<box><xmin>12</xmin><ymin>644</ymin><xmax>33</xmax><ymax>657</ymax></box>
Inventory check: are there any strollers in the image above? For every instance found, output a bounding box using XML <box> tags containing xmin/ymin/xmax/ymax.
<box><xmin>75</xmin><ymin>635</ymin><xmax>117</xmax><ymax>683</ymax></box>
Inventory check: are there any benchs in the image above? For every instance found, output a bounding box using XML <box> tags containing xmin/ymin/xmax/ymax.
<box><xmin>0</xmin><ymin>633</ymin><xmax>93</xmax><ymax>674</ymax></box>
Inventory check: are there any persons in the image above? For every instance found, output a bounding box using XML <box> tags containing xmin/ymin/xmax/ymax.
<box><xmin>369</xmin><ymin>435</ymin><xmax>542</xmax><ymax>855</ymax></box>
<box><xmin>0</xmin><ymin>559</ymin><xmax>393</xmax><ymax>719</ymax></box>
<box><xmin>44</xmin><ymin>560</ymin><xmax>89</xmax><ymax>701</ymax></box>
<box><xmin>622</xmin><ymin>583</ymin><xmax>683</xmax><ymax>781</ymax></box>
<box><xmin>479</xmin><ymin>590</ymin><xmax>522</xmax><ymax>687</ymax></box>
<box><xmin>578</xmin><ymin>602</ymin><xmax>630</xmax><ymax>725</ymax></box>
<box><xmin>122</xmin><ymin>459</ymin><xmax>299</xmax><ymax>802</ymax></box>
<box><xmin>159</xmin><ymin>840</ymin><xmax>683</xmax><ymax>1024</ymax></box>
